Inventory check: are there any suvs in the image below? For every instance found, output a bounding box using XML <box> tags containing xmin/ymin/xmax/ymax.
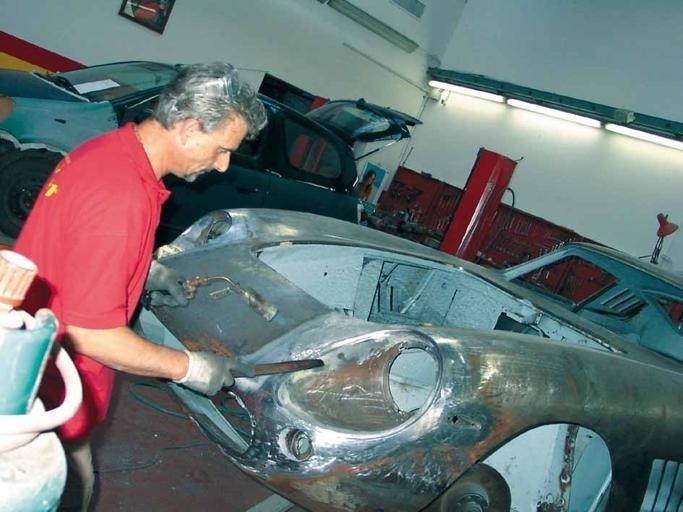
<box><xmin>1</xmin><ymin>58</ymin><xmax>424</xmax><ymax>272</ymax></box>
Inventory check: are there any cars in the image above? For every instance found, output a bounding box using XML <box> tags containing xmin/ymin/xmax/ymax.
<box><xmin>131</xmin><ymin>198</ymin><xmax>682</xmax><ymax>510</ymax></box>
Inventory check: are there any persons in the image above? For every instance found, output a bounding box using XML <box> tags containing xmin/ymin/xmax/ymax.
<box><xmin>12</xmin><ymin>56</ymin><xmax>270</xmax><ymax>512</ymax></box>
<box><xmin>357</xmin><ymin>169</ymin><xmax>376</xmax><ymax>202</ymax></box>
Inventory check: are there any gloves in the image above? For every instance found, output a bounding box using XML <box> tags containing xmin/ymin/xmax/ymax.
<box><xmin>141</xmin><ymin>259</ymin><xmax>189</xmax><ymax>307</ymax></box>
<box><xmin>172</xmin><ymin>349</ymin><xmax>256</xmax><ymax>396</ymax></box>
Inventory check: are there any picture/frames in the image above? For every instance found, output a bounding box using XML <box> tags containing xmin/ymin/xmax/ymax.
<box><xmin>117</xmin><ymin>0</ymin><xmax>177</xmax><ymax>36</ymax></box>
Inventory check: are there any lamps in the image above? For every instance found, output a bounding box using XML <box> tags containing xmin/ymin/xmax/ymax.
<box><xmin>651</xmin><ymin>212</ymin><xmax>678</xmax><ymax>266</ymax></box>
